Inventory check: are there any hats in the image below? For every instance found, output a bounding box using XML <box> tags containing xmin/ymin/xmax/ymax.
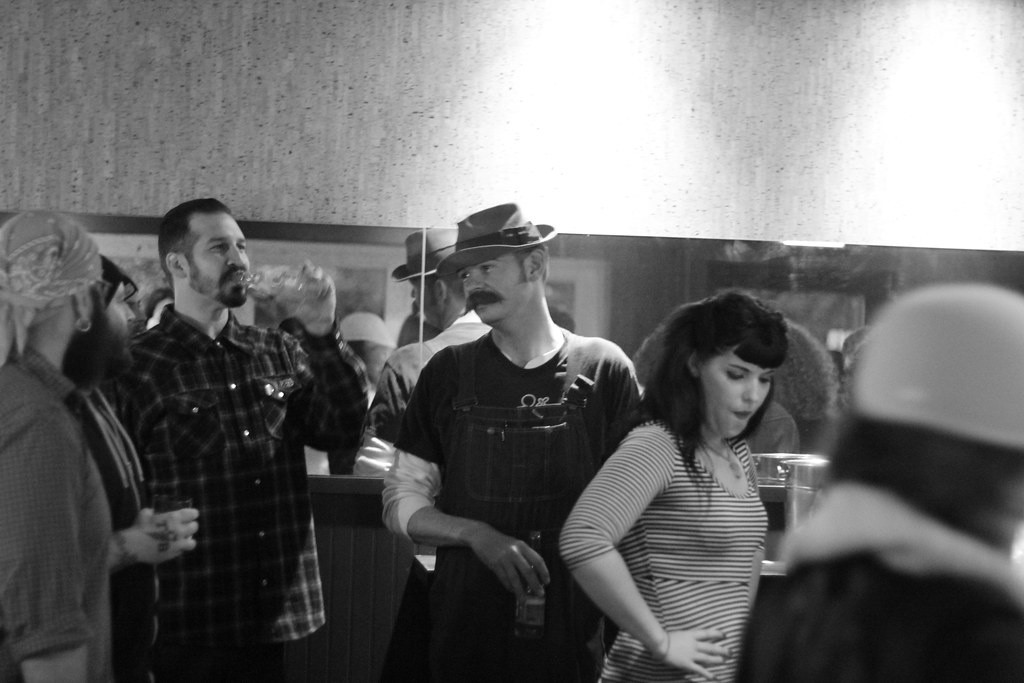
<box><xmin>390</xmin><ymin>228</ymin><xmax>456</xmax><ymax>283</ymax></box>
<box><xmin>851</xmin><ymin>284</ymin><xmax>1023</xmax><ymax>450</ymax></box>
<box><xmin>437</xmin><ymin>203</ymin><xmax>557</xmax><ymax>280</ymax></box>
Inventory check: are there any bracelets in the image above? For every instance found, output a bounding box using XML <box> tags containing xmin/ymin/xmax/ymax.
<box><xmin>653</xmin><ymin>631</ymin><xmax>670</xmax><ymax>662</ymax></box>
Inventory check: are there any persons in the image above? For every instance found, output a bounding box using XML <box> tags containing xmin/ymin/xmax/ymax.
<box><xmin>276</xmin><ymin>319</ymin><xmax>309</xmax><ymax>347</ymax></box>
<box><xmin>64</xmin><ymin>254</ymin><xmax>199</xmax><ymax>683</ymax></box>
<box><xmin>347</xmin><ymin>229</ymin><xmax>494</xmax><ymax>477</ymax></box>
<box><xmin>0</xmin><ymin>209</ymin><xmax>114</xmax><ymax>683</ymax></box>
<box><xmin>326</xmin><ymin>311</ymin><xmax>398</xmax><ymax>475</ymax></box>
<box><xmin>557</xmin><ymin>288</ymin><xmax>790</xmax><ymax>683</ymax></box>
<box><xmin>380</xmin><ymin>204</ymin><xmax>646</xmax><ymax>683</ymax></box>
<box><xmin>95</xmin><ymin>197</ymin><xmax>370</xmax><ymax>683</ymax></box>
<box><xmin>734</xmin><ymin>281</ymin><xmax>1024</xmax><ymax>683</ymax></box>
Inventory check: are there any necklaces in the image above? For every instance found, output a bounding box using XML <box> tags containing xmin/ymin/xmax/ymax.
<box><xmin>698</xmin><ymin>436</ymin><xmax>742</xmax><ymax>479</ymax></box>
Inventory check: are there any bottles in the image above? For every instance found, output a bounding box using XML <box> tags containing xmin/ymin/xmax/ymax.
<box><xmin>237</xmin><ymin>266</ymin><xmax>331</xmax><ymax>299</ymax></box>
<box><xmin>512</xmin><ymin>530</ymin><xmax>549</xmax><ymax>638</ymax></box>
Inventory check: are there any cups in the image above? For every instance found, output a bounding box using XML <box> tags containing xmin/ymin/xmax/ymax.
<box><xmin>151</xmin><ymin>494</ymin><xmax>194</xmax><ymax>520</ymax></box>
